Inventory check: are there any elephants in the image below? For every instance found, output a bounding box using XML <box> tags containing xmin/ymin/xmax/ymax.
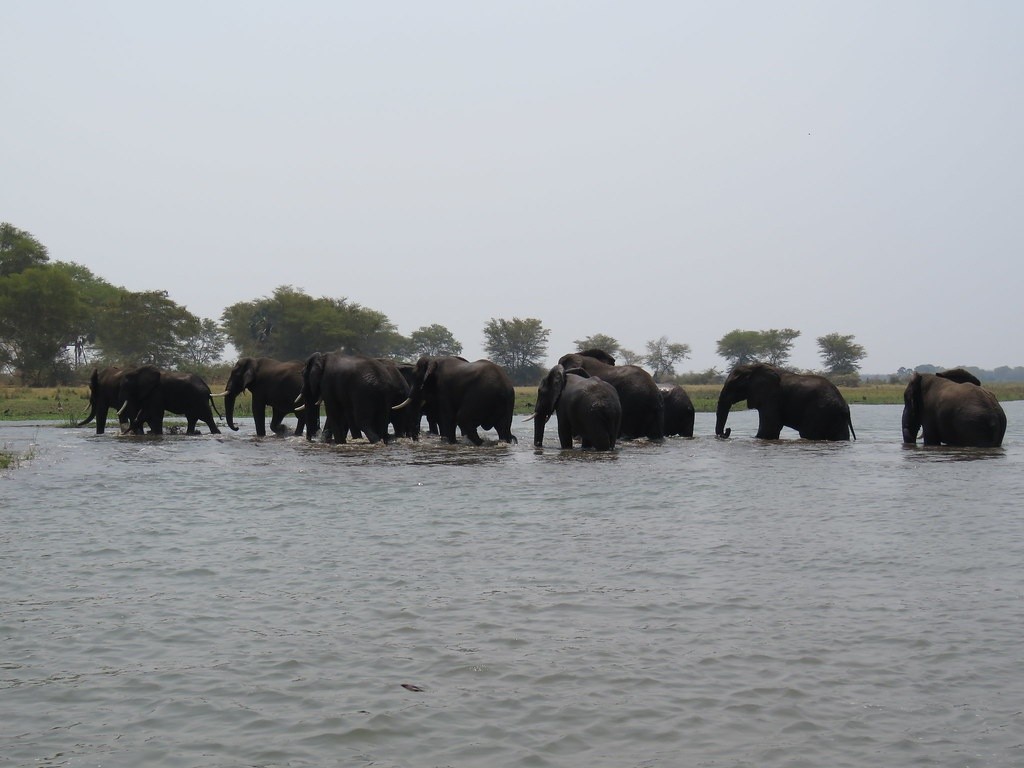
<box><xmin>76</xmin><ymin>349</ymin><xmax>1007</xmax><ymax>452</ymax></box>
